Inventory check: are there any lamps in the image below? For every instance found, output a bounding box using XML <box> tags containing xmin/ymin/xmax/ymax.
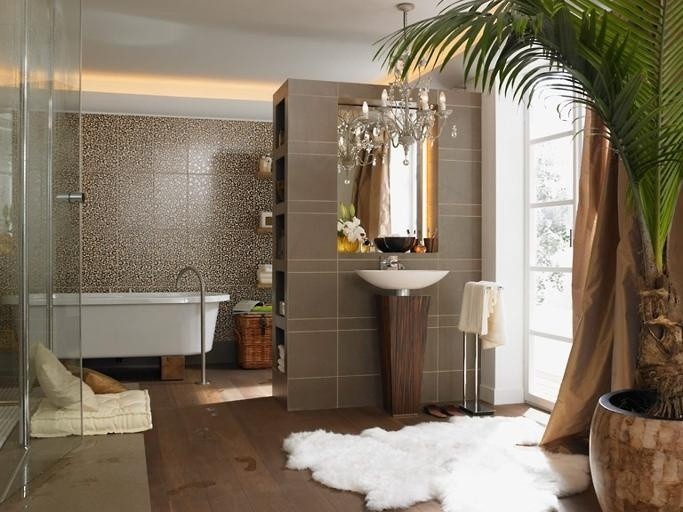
<box><xmin>338</xmin><ymin>114</ymin><xmax>377</xmax><ymax>186</ymax></box>
<box><xmin>352</xmin><ymin>0</ymin><xmax>458</xmax><ymax>167</ymax></box>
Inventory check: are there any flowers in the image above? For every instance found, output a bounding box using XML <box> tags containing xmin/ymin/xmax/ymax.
<box><xmin>343</xmin><ymin>190</ymin><xmax>367</xmax><ymax>244</ymax></box>
<box><xmin>337</xmin><ymin>200</ymin><xmax>348</xmax><ymax>236</ymax></box>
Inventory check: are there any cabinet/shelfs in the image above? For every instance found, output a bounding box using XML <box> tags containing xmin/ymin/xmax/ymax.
<box><xmin>256</xmin><ymin>168</ymin><xmax>274</xmax><ymax>291</ymax></box>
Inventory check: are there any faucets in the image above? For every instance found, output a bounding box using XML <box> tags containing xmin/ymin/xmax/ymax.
<box><xmin>388</xmin><ymin>259</ymin><xmax>406</xmax><ymax>269</ymax></box>
<box><xmin>175</xmin><ymin>266</ymin><xmax>209</xmax><ymax>384</ymax></box>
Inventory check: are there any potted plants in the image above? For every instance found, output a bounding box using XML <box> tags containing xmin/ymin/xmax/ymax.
<box><xmin>370</xmin><ymin>1</ymin><xmax>683</xmax><ymax>512</ymax></box>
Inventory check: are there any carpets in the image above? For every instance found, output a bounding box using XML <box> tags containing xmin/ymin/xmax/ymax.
<box><xmin>282</xmin><ymin>416</ymin><xmax>591</xmax><ymax>512</ymax></box>
<box><xmin>1</xmin><ymin>380</ymin><xmax>152</xmax><ymax>511</ymax></box>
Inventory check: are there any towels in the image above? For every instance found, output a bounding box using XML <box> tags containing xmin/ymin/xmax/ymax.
<box><xmin>257</xmin><ymin>264</ymin><xmax>272</xmax><ymax>285</ymax></box>
<box><xmin>457</xmin><ymin>281</ymin><xmax>519</xmax><ymax>351</ymax></box>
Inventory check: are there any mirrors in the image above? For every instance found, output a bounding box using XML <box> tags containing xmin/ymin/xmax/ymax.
<box><xmin>337</xmin><ymin>102</ymin><xmax>429</xmax><ymax>256</ymax></box>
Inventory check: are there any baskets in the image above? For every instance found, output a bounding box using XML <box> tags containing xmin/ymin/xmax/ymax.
<box><xmin>233</xmin><ymin>313</ymin><xmax>273</xmax><ymax>370</ymax></box>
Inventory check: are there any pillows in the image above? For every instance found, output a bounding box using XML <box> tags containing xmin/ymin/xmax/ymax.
<box><xmin>29</xmin><ymin>340</ymin><xmax>154</xmax><ymax>439</ymax></box>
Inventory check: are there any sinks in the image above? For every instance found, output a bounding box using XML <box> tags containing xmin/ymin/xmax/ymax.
<box><xmin>354</xmin><ymin>270</ymin><xmax>449</xmax><ymax>289</ymax></box>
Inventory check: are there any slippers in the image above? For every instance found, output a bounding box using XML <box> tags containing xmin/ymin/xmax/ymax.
<box><xmin>423</xmin><ymin>403</ymin><xmax>464</xmax><ymax>419</ymax></box>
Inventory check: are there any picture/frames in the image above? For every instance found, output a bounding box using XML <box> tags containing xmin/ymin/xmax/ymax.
<box><xmin>261</xmin><ymin>212</ymin><xmax>272</xmax><ymax>229</ymax></box>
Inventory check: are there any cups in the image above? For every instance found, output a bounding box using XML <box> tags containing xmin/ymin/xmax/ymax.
<box><xmin>360</xmin><ymin>241</ymin><xmax>370</xmax><ymax>255</ymax></box>
<box><xmin>408</xmin><ymin>238</ymin><xmax>434</xmax><ymax>253</ymax></box>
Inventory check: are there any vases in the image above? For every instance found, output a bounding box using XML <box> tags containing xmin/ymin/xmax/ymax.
<box><xmin>337</xmin><ymin>237</ymin><xmax>346</xmax><ymax>252</ymax></box>
<box><xmin>344</xmin><ymin>238</ymin><xmax>360</xmax><ymax>253</ymax></box>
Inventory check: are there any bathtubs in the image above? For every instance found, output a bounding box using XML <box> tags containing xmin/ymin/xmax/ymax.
<box><xmin>1</xmin><ymin>291</ymin><xmax>229</xmax><ymax>381</ymax></box>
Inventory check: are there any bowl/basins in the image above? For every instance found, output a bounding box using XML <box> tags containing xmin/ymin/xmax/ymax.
<box><xmin>374</xmin><ymin>237</ymin><xmax>415</xmax><ymax>253</ymax></box>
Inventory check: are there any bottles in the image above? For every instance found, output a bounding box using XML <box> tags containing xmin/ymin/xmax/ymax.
<box><xmin>260</xmin><ymin>154</ymin><xmax>272</xmax><ymax>173</ymax></box>
